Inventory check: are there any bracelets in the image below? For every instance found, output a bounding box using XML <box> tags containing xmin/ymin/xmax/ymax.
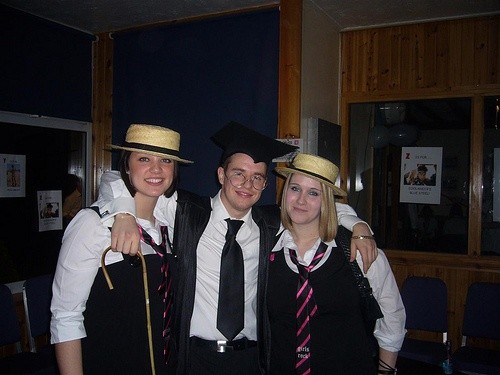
<box><xmin>352</xmin><ymin>236</ymin><xmax>374</xmax><ymax>240</ymax></box>
<box><xmin>379</xmin><ymin>358</ymin><xmax>397</xmax><ymax>375</ymax></box>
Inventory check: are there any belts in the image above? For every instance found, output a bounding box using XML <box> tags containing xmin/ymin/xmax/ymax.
<box><xmin>191</xmin><ymin>337</ymin><xmax>258</xmax><ymax>354</ymax></box>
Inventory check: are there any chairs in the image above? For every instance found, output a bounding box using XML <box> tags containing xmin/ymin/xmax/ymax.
<box><xmin>23</xmin><ymin>273</ymin><xmax>56</xmax><ymax>360</ymax></box>
<box><xmin>450</xmin><ymin>281</ymin><xmax>500</xmax><ymax>375</ymax></box>
<box><xmin>0</xmin><ymin>283</ymin><xmax>61</xmax><ymax>375</ymax></box>
<box><xmin>396</xmin><ymin>276</ymin><xmax>449</xmax><ymax>375</ymax></box>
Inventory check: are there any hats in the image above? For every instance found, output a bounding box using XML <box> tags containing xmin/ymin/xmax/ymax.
<box><xmin>418</xmin><ymin>163</ymin><xmax>428</xmax><ymax>171</ymax></box>
<box><xmin>211</xmin><ymin>121</ymin><xmax>299</xmax><ymax>166</ymax></box>
<box><xmin>104</xmin><ymin>124</ymin><xmax>194</xmax><ymax>165</ymax></box>
<box><xmin>274</xmin><ymin>153</ymin><xmax>348</xmax><ymax>199</ymax></box>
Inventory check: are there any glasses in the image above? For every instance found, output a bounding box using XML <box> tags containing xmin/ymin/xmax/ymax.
<box><xmin>223</xmin><ymin>172</ymin><xmax>271</xmax><ymax>191</ymax></box>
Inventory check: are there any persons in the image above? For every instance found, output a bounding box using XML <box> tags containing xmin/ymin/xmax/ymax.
<box><xmin>7</xmin><ymin>164</ymin><xmax>16</xmax><ymax>187</ymax></box>
<box><xmin>411</xmin><ymin>166</ymin><xmax>432</xmax><ymax>186</ymax></box>
<box><xmin>98</xmin><ymin>121</ymin><xmax>378</xmax><ymax>375</ymax></box>
<box><xmin>40</xmin><ymin>203</ymin><xmax>56</xmax><ymax>219</ymax></box>
<box><xmin>49</xmin><ymin>124</ymin><xmax>194</xmax><ymax>375</ymax></box>
<box><xmin>270</xmin><ymin>153</ymin><xmax>408</xmax><ymax>375</ymax></box>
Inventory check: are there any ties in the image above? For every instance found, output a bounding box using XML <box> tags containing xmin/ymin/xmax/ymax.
<box><xmin>216</xmin><ymin>218</ymin><xmax>245</xmax><ymax>341</ymax></box>
<box><xmin>289</xmin><ymin>242</ymin><xmax>328</xmax><ymax>375</ymax></box>
<box><xmin>136</xmin><ymin>224</ymin><xmax>175</xmax><ymax>369</ymax></box>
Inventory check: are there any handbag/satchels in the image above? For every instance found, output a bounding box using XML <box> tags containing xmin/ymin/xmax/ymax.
<box><xmin>335</xmin><ymin>231</ymin><xmax>383</xmax><ymax>349</ymax></box>
